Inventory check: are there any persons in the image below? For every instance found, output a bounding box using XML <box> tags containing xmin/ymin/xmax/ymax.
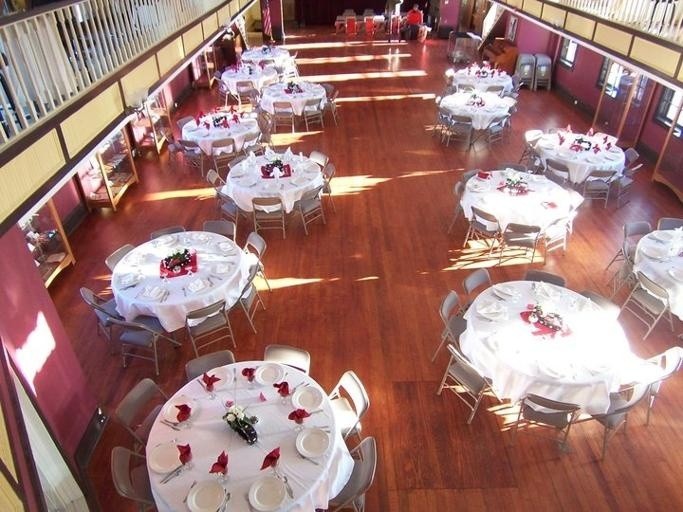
<box><xmin>385</xmin><ymin>0</ymin><xmax>422</xmax><ymax>42</ymax></box>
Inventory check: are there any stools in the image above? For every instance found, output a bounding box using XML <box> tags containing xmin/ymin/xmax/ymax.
<box><xmin>514</xmin><ymin>52</ymin><xmax>555</xmax><ymax>93</ymax></box>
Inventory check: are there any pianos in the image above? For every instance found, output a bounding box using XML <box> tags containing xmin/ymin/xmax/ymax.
<box><xmin>482</xmin><ymin>38</ymin><xmax>518</xmax><ymax>76</ymax></box>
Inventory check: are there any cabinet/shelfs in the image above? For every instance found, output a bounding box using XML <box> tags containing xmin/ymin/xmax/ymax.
<box><xmin>79</xmin><ymin>126</ymin><xmax>140</xmax><ymax>213</ymax></box>
<box><xmin>191</xmin><ymin>46</ymin><xmax>219</xmax><ymax>90</ymax></box>
<box><xmin>126</xmin><ymin>92</ymin><xmax>174</xmax><ymax>158</ymax></box>
<box><xmin>21</xmin><ymin>196</ymin><xmax>76</xmax><ymax>288</ymax></box>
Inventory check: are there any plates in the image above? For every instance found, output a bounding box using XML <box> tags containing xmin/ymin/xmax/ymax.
<box><xmin>186</xmin><ymin>44</ymin><xmax>322</xmax><ymax>140</ymax></box>
<box><xmin>114</xmin><ymin>232</ymin><xmax>239</xmax><ymax>305</ymax></box>
<box><xmin>230</xmin><ymin>155</ymin><xmax>320</xmax><ymax>188</ymax></box>
<box><xmin>639</xmin><ymin>226</ymin><xmax>682</xmax><ymax>283</ymax></box>
<box><xmin>481</xmin><ymin>280</ymin><xmax>619</xmax><ymax>381</ymax></box>
<box><xmin>146</xmin><ymin>362</ymin><xmax>330</xmax><ymax>511</ymax></box>
<box><xmin>467</xmin><ymin>134</ymin><xmax>620</xmax><ymax>226</ymax></box>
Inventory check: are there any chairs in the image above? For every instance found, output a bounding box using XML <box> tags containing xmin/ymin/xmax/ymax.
<box><xmin>110</xmin><ymin>343</ymin><xmax>378</xmax><ymax>511</ymax></box>
<box><xmin>333</xmin><ymin>7</ymin><xmax>404</xmax><ymax>39</ymax></box>
<box><xmin>206</xmin><ymin>148</ymin><xmax>338</xmax><ymax>241</ymax></box>
<box><xmin>430</xmin><ymin>266</ymin><xmax>682</xmax><ymax>465</ymax></box>
<box><xmin>444</xmin><ymin>163</ymin><xmax>584</xmax><ymax>272</ymax></box>
<box><xmin>602</xmin><ymin>216</ymin><xmax>682</xmax><ymax>343</ymax></box>
<box><xmin>208</xmin><ymin>45</ymin><xmax>342</xmax><ymax>135</ymax></box>
<box><xmin>519</xmin><ymin>124</ymin><xmax>644</xmax><ymax>209</ymax></box>
<box><xmin>160</xmin><ymin>103</ymin><xmax>275</xmax><ymax>178</ymax></box>
<box><xmin>80</xmin><ymin>219</ymin><xmax>272</xmax><ymax>377</ymax></box>
<box><xmin>431</xmin><ymin>67</ymin><xmax>523</xmax><ymax>152</ymax></box>
